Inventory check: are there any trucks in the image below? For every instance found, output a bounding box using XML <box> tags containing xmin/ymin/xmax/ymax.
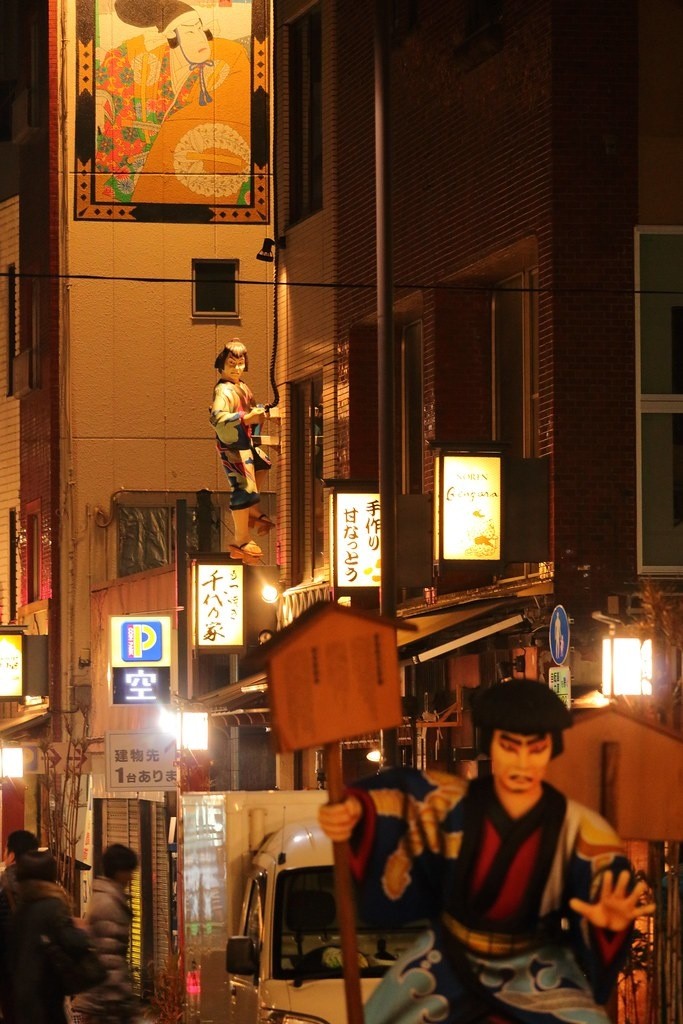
<box><xmin>175</xmin><ymin>789</ymin><xmax>434</xmax><ymax>1024</ymax></box>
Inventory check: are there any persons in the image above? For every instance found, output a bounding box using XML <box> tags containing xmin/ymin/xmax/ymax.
<box><xmin>210</xmin><ymin>338</ymin><xmax>274</xmax><ymax>563</ymax></box>
<box><xmin>0</xmin><ymin>830</ymin><xmax>69</xmax><ymax>1024</ymax></box>
<box><xmin>319</xmin><ymin>678</ymin><xmax>655</xmax><ymax>1024</ymax></box>
<box><xmin>72</xmin><ymin>844</ymin><xmax>138</xmax><ymax>1024</ymax></box>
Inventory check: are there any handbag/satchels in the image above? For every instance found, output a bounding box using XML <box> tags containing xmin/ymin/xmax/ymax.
<box><xmin>44</xmin><ymin>943</ymin><xmax>108</xmax><ymax>994</ymax></box>
